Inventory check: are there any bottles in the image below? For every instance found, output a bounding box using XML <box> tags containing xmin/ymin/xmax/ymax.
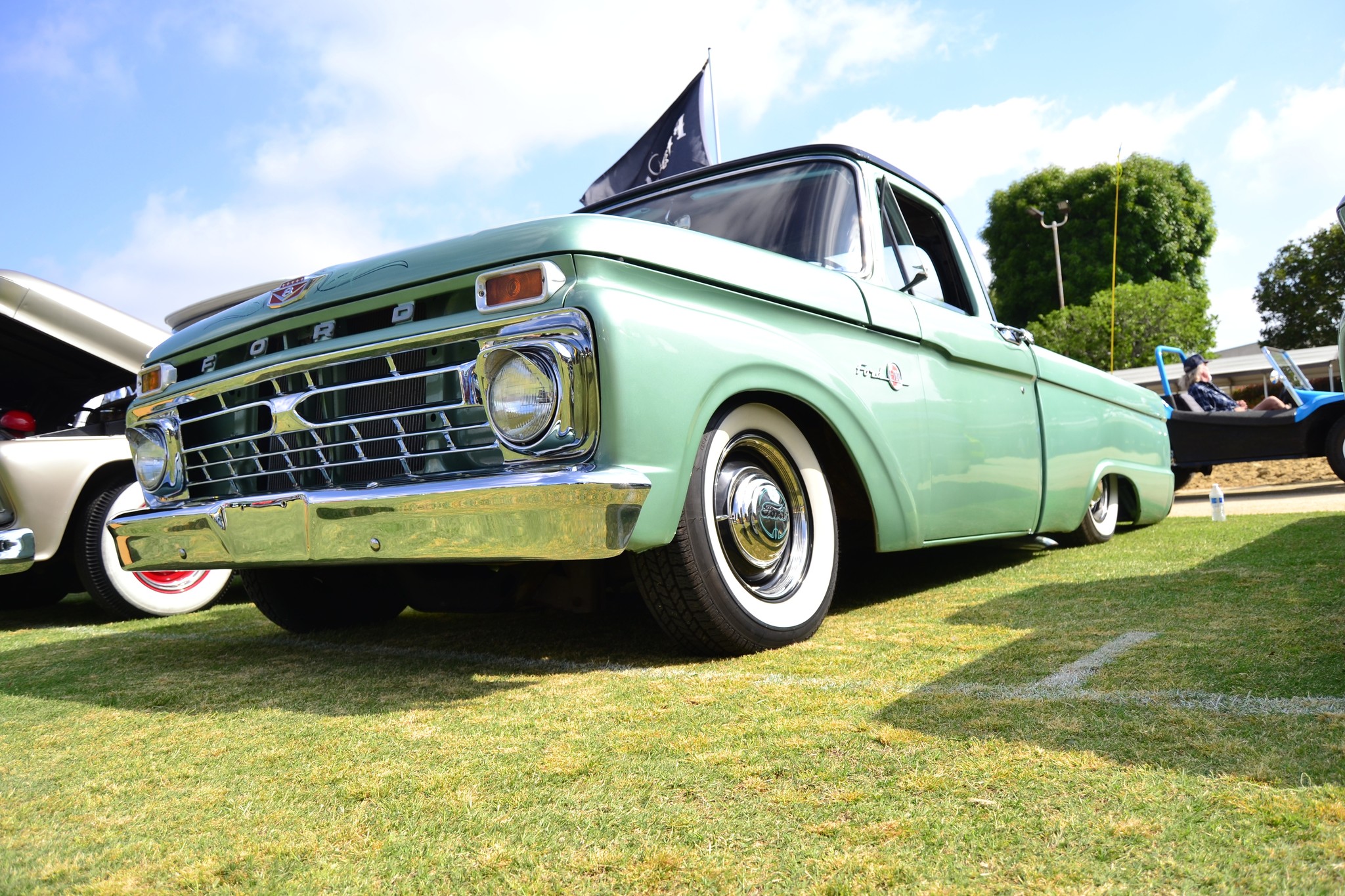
<box><xmin>1208</xmin><ymin>483</ymin><xmax>1226</xmax><ymax>522</ymax></box>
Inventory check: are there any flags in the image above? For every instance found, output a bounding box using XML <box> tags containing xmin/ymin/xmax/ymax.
<box><xmin>580</xmin><ymin>59</ymin><xmax>714</xmax><ymax>207</ymax></box>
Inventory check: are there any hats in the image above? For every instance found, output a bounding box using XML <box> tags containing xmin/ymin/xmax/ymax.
<box><xmin>1183</xmin><ymin>353</ymin><xmax>1209</xmax><ymax>372</ymax></box>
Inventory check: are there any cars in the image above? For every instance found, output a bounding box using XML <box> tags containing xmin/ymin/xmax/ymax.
<box><xmin>1150</xmin><ymin>342</ymin><xmax>1345</xmax><ymax>492</ymax></box>
<box><xmin>103</xmin><ymin>137</ymin><xmax>1161</xmax><ymax>667</ymax></box>
<box><xmin>0</xmin><ymin>266</ymin><xmax>236</xmax><ymax>622</ymax></box>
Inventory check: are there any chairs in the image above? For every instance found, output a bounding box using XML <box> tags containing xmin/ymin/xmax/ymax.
<box><xmin>1177</xmin><ymin>392</ymin><xmax>1203</xmax><ymax>412</ymax></box>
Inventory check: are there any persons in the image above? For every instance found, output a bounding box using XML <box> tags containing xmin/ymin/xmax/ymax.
<box><xmin>1179</xmin><ymin>354</ymin><xmax>1290</xmax><ymax>412</ymax></box>
